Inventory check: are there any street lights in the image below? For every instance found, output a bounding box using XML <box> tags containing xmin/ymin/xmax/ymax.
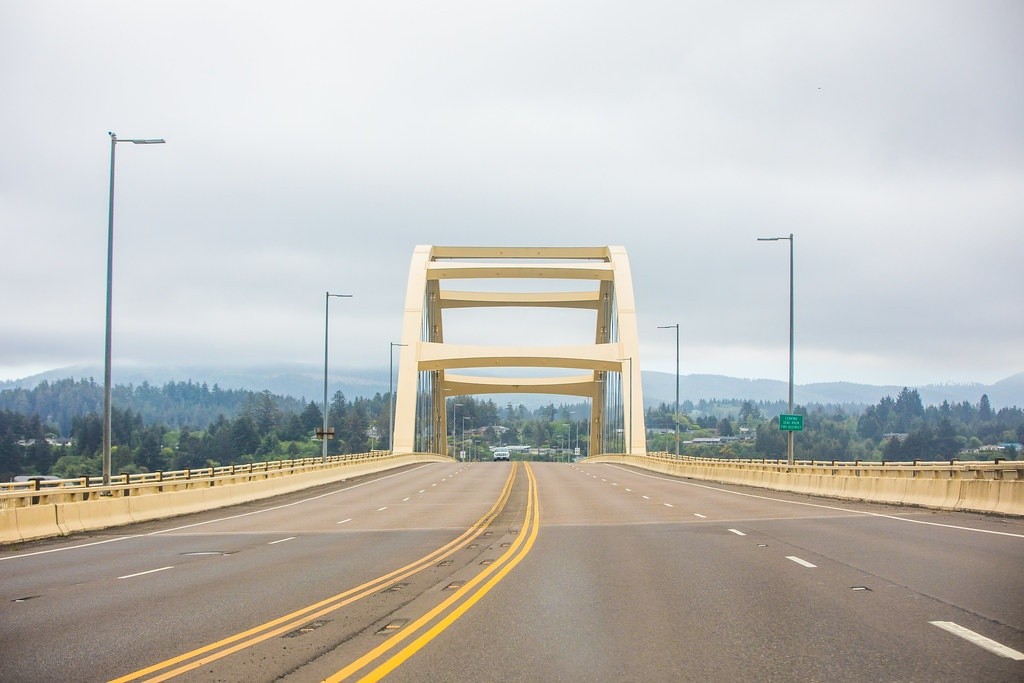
<box><xmin>656</xmin><ymin>322</ymin><xmax>680</xmax><ymax>459</ymax></box>
<box><xmin>388</xmin><ymin>342</ymin><xmax>409</xmax><ymax>452</ymax></box>
<box><xmin>756</xmin><ymin>233</ymin><xmax>795</xmax><ymax>466</ymax></box>
<box><xmin>616</xmin><ymin>357</ymin><xmax>633</xmax><ymax>453</ymax></box>
<box><xmin>569</xmin><ymin>410</ymin><xmax>579</xmax><ymax>460</ymax></box>
<box><xmin>320</xmin><ymin>291</ymin><xmax>353</xmax><ymax>462</ymax></box>
<box><xmin>467</xmin><ymin>428</ymin><xmax>476</xmax><ymax>462</ymax></box>
<box><xmin>461</xmin><ymin>416</ymin><xmax>471</xmax><ymax>462</ymax></box>
<box><xmin>556</xmin><ymin>434</ymin><xmax>564</xmax><ymax>463</ymax></box>
<box><xmin>453</xmin><ymin>402</ymin><xmax>463</xmax><ymax>457</ymax></box>
<box><xmin>562</xmin><ymin>423</ymin><xmax>571</xmax><ymax>463</ymax></box>
<box><xmin>438</xmin><ymin>386</ymin><xmax>452</xmax><ymax>452</ymax></box>
<box><xmin>594</xmin><ymin>379</ymin><xmax>608</xmax><ymax>452</ymax></box>
<box><xmin>99</xmin><ymin>131</ymin><xmax>167</xmax><ymax>485</ymax></box>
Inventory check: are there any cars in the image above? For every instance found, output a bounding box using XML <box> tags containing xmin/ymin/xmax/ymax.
<box><xmin>492</xmin><ymin>447</ymin><xmax>511</xmax><ymax>461</ymax></box>
